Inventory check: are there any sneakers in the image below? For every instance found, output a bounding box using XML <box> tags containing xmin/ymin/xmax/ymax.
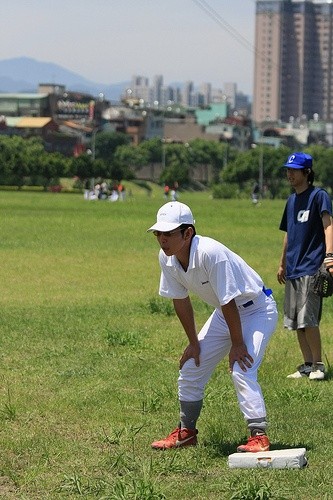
<box><xmin>287</xmin><ymin>364</ymin><xmax>311</xmax><ymax>378</ymax></box>
<box><xmin>236</xmin><ymin>435</ymin><xmax>270</xmax><ymax>452</ymax></box>
<box><xmin>151</xmin><ymin>427</ymin><xmax>199</xmax><ymax>449</ymax></box>
<box><xmin>309</xmin><ymin>363</ymin><xmax>324</xmax><ymax>379</ymax></box>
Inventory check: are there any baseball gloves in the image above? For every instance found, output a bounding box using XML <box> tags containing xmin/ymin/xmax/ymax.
<box><xmin>311</xmin><ymin>268</ymin><xmax>333</xmax><ymax>297</ymax></box>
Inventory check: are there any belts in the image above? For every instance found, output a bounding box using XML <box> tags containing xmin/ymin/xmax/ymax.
<box><xmin>239</xmin><ymin>286</ymin><xmax>267</xmax><ymax>309</ymax></box>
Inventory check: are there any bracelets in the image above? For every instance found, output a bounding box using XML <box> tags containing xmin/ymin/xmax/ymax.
<box><xmin>323</xmin><ymin>257</ymin><xmax>333</xmax><ymax>263</ymax></box>
<box><xmin>326</xmin><ymin>253</ymin><xmax>333</xmax><ymax>257</ymax></box>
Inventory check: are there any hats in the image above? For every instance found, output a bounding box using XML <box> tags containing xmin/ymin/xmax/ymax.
<box><xmin>282</xmin><ymin>152</ymin><xmax>313</xmax><ymax>169</ymax></box>
<box><xmin>146</xmin><ymin>201</ymin><xmax>195</xmax><ymax>232</ymax></box>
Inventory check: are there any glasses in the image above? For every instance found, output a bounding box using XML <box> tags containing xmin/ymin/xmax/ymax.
<box><xmin>153</xmin><ymin>228</ymin><xmax>186</xmax><ymax>237</ymax></box>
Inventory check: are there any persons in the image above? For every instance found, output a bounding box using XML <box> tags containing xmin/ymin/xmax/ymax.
<box><xmin>146</xmin><ymin>201</ymin><xmax>278</xmax><ymax>452</ymax></box>
<box><xmin>277</xmin><ymin>152</ymin><xmax>333</xmax><ymax>380</ymax></box>
<box><xmin>164</xmin><ymin>181</ymin><xmax>179</xmax><ymax>201</ymax></box>
<box><xmin>84</xmin><ymin>181</ymin><xmax>125</xmax><ymax>201</ymax></box>
